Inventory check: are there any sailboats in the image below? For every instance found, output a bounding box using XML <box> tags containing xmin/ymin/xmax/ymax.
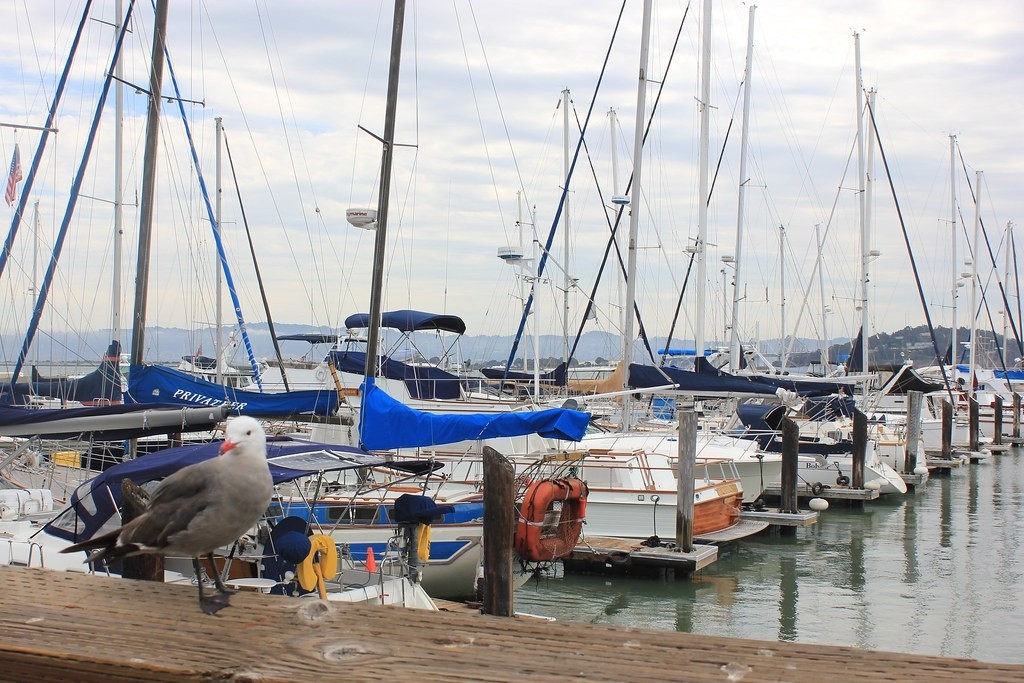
<box><xmin>0</xmin><ymin>0</ymin><xmax>1023</xmax><ymax>621</ymax></box>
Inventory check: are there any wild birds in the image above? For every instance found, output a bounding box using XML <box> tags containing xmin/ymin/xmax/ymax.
<box><xmin>84</xmin><ymin>416</ymin><xmax>274</xmax><ymax>616</ymax></box>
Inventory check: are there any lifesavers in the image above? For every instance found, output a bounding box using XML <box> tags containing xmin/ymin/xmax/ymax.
<box><xmin>812</xmin><ymin>483</ymin><xmax>824</xmax><ymax>495</ymax></box>
<box><xmin>515</xmin><ymin>479</ymin><xmax>586</xmax><ymax>561</ymax></box>
<box><xmin>608</xmin><ymin>551</ymin><xmax>631</xmax><ymax>566</ymax></box>
<box><xmin>316</xmin><ymin>370</ymin><xmax>326</xmax><ymax>381</ymax></box>
<box><xmin>837</xmin><ymin>477</ymin><xmax>849</xmax><ymax>486</ymax></box>
<box><xmin>296</xmin><ymin>534</ymin><xmax>337</xmax><ymax>592</ymax></box>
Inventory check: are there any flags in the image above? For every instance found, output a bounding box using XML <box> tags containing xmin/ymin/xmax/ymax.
<box><xmin>5</xmin><ymin>145</ymin><xmax>22</xmax><ymax>207</ymax></box>
